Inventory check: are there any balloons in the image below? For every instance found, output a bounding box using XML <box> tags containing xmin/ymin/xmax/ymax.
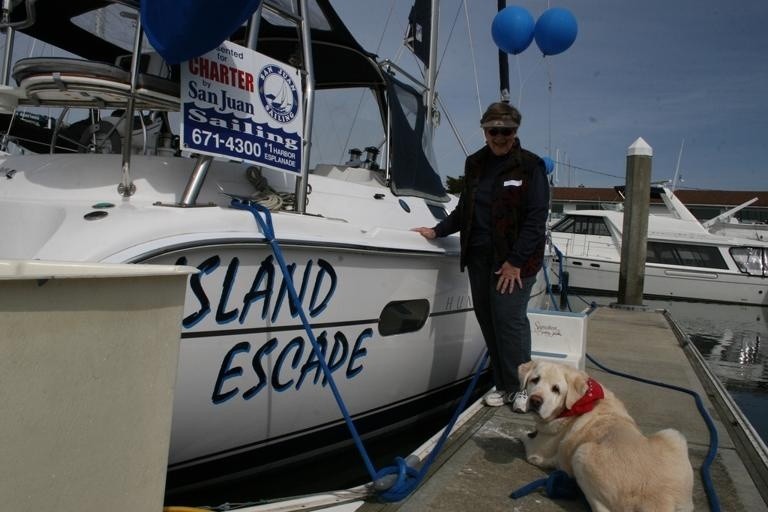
<box><xmin>490</xmin><ymin>3</ymin><xmax>535</xmax><ymax>53</ymax></box>
<box><xmin>533</xmin><ymin>6</ymin><xmax>579</xmax><ymax>58</ymax></box>
<box><xmin>541</xmin><ymin>155</ymin><xmax>554</xmax><ymax>175</ymax></box>
<box><xmin>140</xmin><ymin>0</ymin><xmax>261</xmax><ymax>65</ymax></box>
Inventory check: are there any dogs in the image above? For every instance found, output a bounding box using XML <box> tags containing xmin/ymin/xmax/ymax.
<box><xmin>517</xmin><ymin>358</ymin><xmax>695</xmax><ymax>512</ymax></box>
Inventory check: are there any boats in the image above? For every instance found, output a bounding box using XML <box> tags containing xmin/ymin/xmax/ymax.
<box><xmin>544</xmin><ymin>183</ymin><xmax>768</xmax><ymax>306</ymax></box>
<box><xmin>1</xmin><ymin>1</ymin><xmax>555</xmax><ymax>472</ymax></box>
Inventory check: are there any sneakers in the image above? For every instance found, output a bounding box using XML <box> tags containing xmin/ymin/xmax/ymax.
<box><xmin>484</xmin><ymin>390</ymin><xmax>514</xmax><ymax>406</ymax></box>
<box><xmin>513</xmin><ymin>389</ymin><xmax>531</xmax><ymax>413</ymax></box>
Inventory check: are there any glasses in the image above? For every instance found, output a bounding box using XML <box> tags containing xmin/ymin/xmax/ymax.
<box><xmin>488</xmin><ymin>128</ymin><xmax>513</xmax><ymax>135</ymax></box>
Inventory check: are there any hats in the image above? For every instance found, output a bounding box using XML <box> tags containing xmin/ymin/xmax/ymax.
<box><xmin>480</xmin><ymin>114</ymin><xmax>519</xmax><ymax>128</ymax></box>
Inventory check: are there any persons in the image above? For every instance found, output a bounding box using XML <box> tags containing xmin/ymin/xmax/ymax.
<box><xmin>410</xmin><ymin>101</ymin><xmax>551</xmax><ymax>413</ymax></box>
